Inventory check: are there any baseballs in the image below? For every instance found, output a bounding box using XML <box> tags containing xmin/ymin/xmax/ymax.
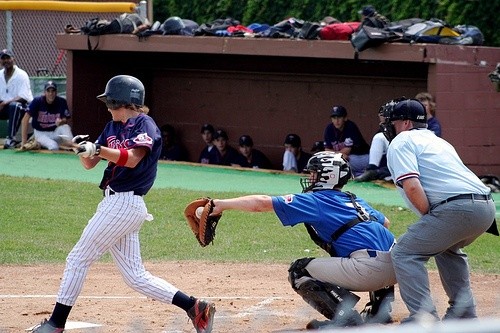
<box><xmin>195</xmin><ymin>206</ymin><xmax>205</xmax><ymax>219</ymax></box>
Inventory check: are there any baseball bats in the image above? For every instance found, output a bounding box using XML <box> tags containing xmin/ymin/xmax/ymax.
<box><xmin>54</xmin><ymin>134</ymin><xmax>81</xmax><ymax>148</ymax></box>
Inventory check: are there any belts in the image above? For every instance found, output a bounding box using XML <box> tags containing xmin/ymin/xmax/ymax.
<box><xmin>442</xmin><ymin>193</ymin><xmax>493</xmax><ymax>201</ymax></box>
<box><xmin>104</xmin><ymin>189</ymin><xmax>142</xmax><ymax>197</ymax></box>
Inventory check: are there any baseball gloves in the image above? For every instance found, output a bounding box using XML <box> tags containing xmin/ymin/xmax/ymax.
<box><xmin>184</xmin><ymin>197</ymin><xmax>222</xmax><ymax>247</ymax></box>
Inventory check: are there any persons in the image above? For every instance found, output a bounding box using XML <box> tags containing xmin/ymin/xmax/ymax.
<box><xmin>184</xmin><ymin>150</ymin><xmax>399</xmax><ymax>328</ymax></box>
<box><xmin>327</xmin><ymin>101</ymin><xmax>372</xmax><ymax>183</ymax></box>
<box><xmin>285</xmin><ymin>131</ymin><xmax>312</xmax><ymax>174</ymax></box>
<box><xmin>197</xmin><ymin>119</ymin><xmax>217</xmax><ymax>164</ymax></box>
<box><xmin>215</xmin><ymin>122</ymin><xmax>235</xmax><ymax>165</ymax></box>
<box><xmin>379</xmin><ymin>95</ymin><xmax>496</xmax><ymax>326</ymax></box>
<box><xmin>26</xmin><ymin>73</ymin><xmax>217</xmax><ymax>332</ymax></box>
<box><xmin>0</xmin><ymin>46</ymin><xmax>34</xmax><ymax>149</ymax></box>
<box><xmin>21</xmin><ymin>80</ymin><xmax>74</xmax><ymax>151</ymax></box>
<box><xmin>235</xmin><ymin>130</ymin><xmax>271</xmax><ymax>171</ymax></box>
<box><xmin>356</xmin><ymin>91</ymin><xmax>444</xmax><ymax>183</ymax></box>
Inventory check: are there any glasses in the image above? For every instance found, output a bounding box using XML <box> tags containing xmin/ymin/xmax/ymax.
<box><xmin>106</xmin><ymin>99</ymin><xmax>121</xmax><ymax>109</ymax></box>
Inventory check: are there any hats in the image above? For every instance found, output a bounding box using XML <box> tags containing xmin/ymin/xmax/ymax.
<box><xmin>239</xmin><ymin>135</ymin><xmax>253</xmax><ymax>146</ymax></box>
<box><xmin>284</xmin><ymin>134</ymin><xmax>301</xmax><ymax>147</ymax></box>
<box><xmin>45</xmin><ymin>81</ymin><xmax>58</xmax><ymax>91</ymax></box>
<box><xmin>201</xmin><ymin>124</ymin><xmax>215</xmax><ymax>134</ymax></box>
<box><xmin>213</xmin><ymin>129</ymin><xmax>229</xmax><ymax>139</ymax></box>
<box><xmin>330</xmin><ymin>106</ymin><xmax>347</xmax><ymax>117</ymax></box>
<box><xmin>1</xmin><ymin>49</ymin><xmax>13</xmax><ymax>58</ymax></box>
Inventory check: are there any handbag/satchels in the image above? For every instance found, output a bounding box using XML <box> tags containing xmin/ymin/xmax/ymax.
<box><xmin>402</xmin><ymin>18</ymin><xmax>484</xmax><ymax>46</ymax></box>
<box><xmin>351</xmin><ymin>27</ymin><xmax>401</xmax><ymax>59</ymax></box>
<box><xmin>81</xmin><ymin>12</ymin><xmax>146</xmax><ymax>51</ymax></box>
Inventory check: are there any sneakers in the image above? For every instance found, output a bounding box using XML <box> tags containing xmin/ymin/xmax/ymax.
<box><xmin>27</xmin><ymin>318</ymin><xmax>65</xmax><ymax>333</ymax></box>
<box><xmin>187</xmin><ymin>296</ymin><xmax>217</xmax><ymax>333</ymax></box>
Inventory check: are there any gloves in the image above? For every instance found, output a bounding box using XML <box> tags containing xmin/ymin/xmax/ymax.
<box><xmin>77</xmin><ymin>141</ymin><xmax>101</xmax><ymax>158</ymax></box>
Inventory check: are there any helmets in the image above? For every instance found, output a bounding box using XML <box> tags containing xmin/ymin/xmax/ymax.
<box><xmin>163</xmin><ymin>17</ymin><xmax>185</xmax><ymax>34</ymax></box>
<box><xmin>98</xmin><ymin>75</ymin><xmax>146</xmax><ymax>106</ymax></box>
<box><xmin>381</xmin><ymin>96</ymin><xmax>427</xmax><ymax>143</ymax></box>
<box><xmin>300</xmin><ymin>151</ymin><xmax>354</xmax><ymax>191</ymax></box>
